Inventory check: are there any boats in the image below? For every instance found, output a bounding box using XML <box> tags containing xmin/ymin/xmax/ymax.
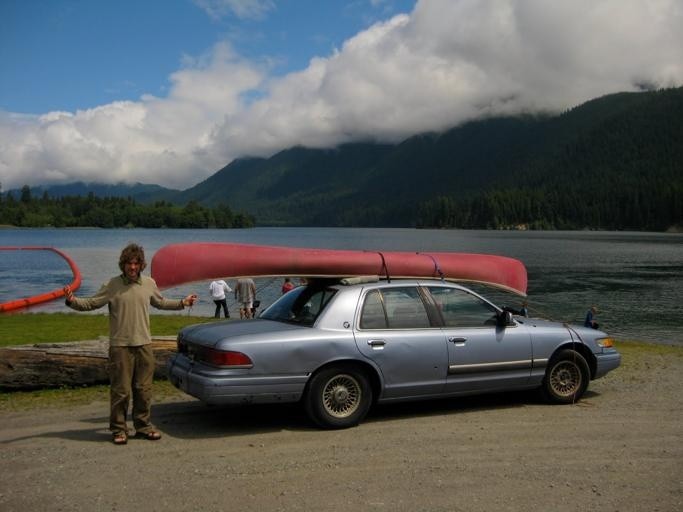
<box><xmin>151</xmin><ymin>241</ymin><xmax>528</xmax><ymax>300</ymax></box>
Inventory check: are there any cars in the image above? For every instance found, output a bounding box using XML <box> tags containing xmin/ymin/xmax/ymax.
<box><xmin>168</xmin><ymin>278</ymin><xmax>621</xmax><ymax>430</ymax></box>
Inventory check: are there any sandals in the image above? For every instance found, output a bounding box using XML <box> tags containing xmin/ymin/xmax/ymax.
<box><xmin>113</xmin><ymin>435</ymin><xmax>127</xmax><ymax>444</ymax></box>
<box><xmin>136</xmin><ymin>431</ymin><xmax>161</xmax><ymax>440</ymax></box>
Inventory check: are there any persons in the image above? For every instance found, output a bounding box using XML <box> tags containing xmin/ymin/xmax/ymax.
<box><xmin>233</xmin><ymin>277</ymin><xmax>257</xmax><ymax>319</ymax></box>
<box><xmin>281</xmin><ymin>277</ymin><xmax>294</xmax><ymax>295</ymax></box>
<box><xmin>521</xmin><ymin>301</ymin><xmax>528</xmax><ymax>316</ymax></box>
<box><xmin>586</xmin><ymin>306</ymin><xmax>599</xmax><ymax>329</ymax></box>
<box><xmin>62</xmin><ymin>244</ymin><xmax>197</xmax><ymax>443</ymax></box>
<box><xmin>298</xmin><ymin>278</ymin><xmax>306</xmax><ymax>285</ymax></box>
<box><xmin>208</xmin><ymin>279</ymin><xmax>233</xmax><ymax>318</ymax></box>
